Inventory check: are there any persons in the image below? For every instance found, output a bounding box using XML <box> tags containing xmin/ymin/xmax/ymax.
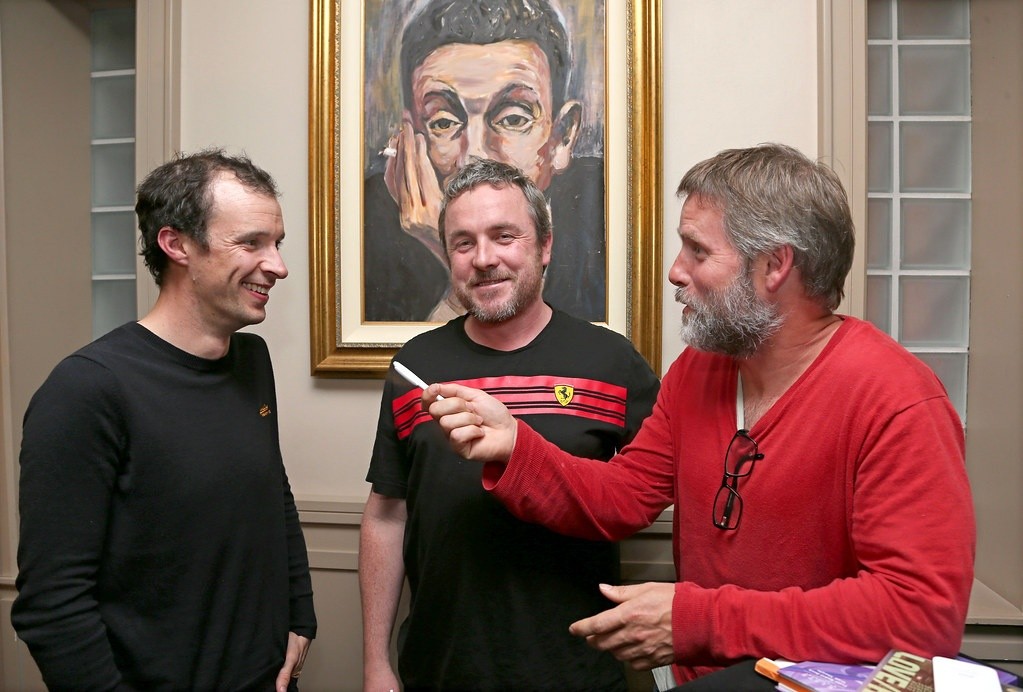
<box><xmin>10</xmin><ymin>150</ymin><xmax>318</xmax><ymax>692</ymax></box>
<box><xmin>421</xmin><ymin>145</ymin><xmax>977</xmax><ymax>687</ymax></box>
<box><xmin>358</xmin><ymin>159</ymin><xmax>662</xmax><ymax>692</ymax></box>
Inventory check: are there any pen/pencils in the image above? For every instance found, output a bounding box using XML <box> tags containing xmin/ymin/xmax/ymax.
<box><xmin>391</xmin><ymin>358</ymin><xmax>445</xmax><ymax>401</ymax></box>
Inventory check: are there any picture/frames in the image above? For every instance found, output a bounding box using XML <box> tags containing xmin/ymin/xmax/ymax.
<box><xmin>308</xmin><ymin>0</ymin><xmax>663</xmax><ymax>380</ymax></box>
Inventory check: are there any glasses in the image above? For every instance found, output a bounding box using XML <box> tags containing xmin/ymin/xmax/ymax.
<box><xmin>712</xmin><ymin>430</ymin><xmax>764</xmax><ymax>530</ymax></box>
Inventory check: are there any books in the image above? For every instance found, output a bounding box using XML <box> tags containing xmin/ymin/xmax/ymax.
<box><xmin>778</xmin><ymin>655</ymin><xmax>1019</xmax><ymax>692</ymax></box>
